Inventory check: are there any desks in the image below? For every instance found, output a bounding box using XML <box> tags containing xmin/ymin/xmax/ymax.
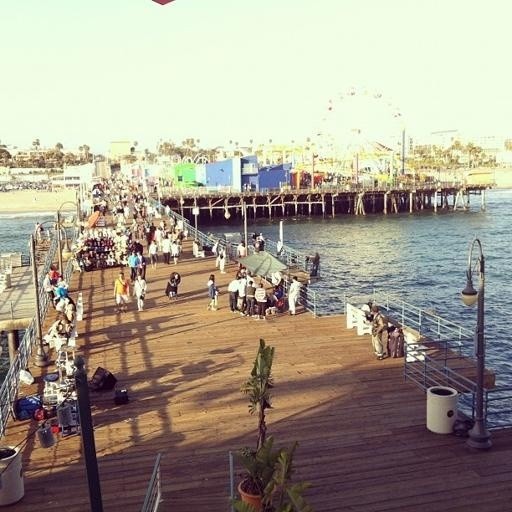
<box><xmin>46</xmin><ymin>320</ymin><xmax>79</xmax><ymax>366</ymax></box>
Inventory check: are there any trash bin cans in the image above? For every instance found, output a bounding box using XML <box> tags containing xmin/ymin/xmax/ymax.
<box><xmin>0</xmin><ymin>445</ymin><xmax>25</xmax><ymax>506</ymax></box>
<box><xmin>426</xmin><ymin>386</ymin><xmax>458</xmax><ymax>435</ymax></box>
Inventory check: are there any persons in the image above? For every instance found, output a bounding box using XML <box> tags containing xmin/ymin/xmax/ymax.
<box><xmin>206</xmin><ymin>275</ymin><xmax>217</xmax><ymax>311</ymax></box>
<box><xmin>166</xmin><ymin>205</ymin><xmax>170</xmax><ymax>217</ymax></box>
<box><xmin>227</xmin><ymin>267</ymin><xmax>283</xmax><ymax>320</ymax></box>
<box><xmin>288</xmin><ymin>276</ymin><xmax>301</xmax><ymax>315</ymax></box>
<box><xmin>166</xmin><ymin>272</ymin><xmax>180</xmax><ymax>300</ymax></box>
<box><xmin>133</xmin><ymin>275</ymin><xmax>147</xmax><ymax>312</ymax></box>
<box><xmin>112</xmin><ymin>272</ymin><xmax>130</xmax><ymax>313</ymax></box>
<box><xmin>96</xmin><ymin>184</ymin><xmax>153</xmax><ymax>224</ymax></box>
<box><xmin>255</xmin><ymin>237</ymin><xmax>264</xmax><ymax>251</ymax></box>
<box><xmin>372</xmin><ymin>307</ymin><xmax>386</xmax><ymax>360</ymax></box>
<box><xmin>42</xmin><ymin>265</ymin><xmax>77</xmax><ymax>323</ymax></box>
<box><xmin>128</xmin><ymin>251</ymin><xmax>146</xmax><ymax>281</ymax></box>
<box><xmin>126</xmin><ymin>215</ymin><xmax>189</xmax><ymax>270</ymax></box>
<box><xmin>237</xmin><ymin>241</ymin><xmax>246</xmax><ymax>269</ymax></box>
<box><xmin>361</xmin><ymin>302</ymin><xmax>374</xmax><ymax>322</ymax></box>
<box><xmin>214</xmin><ymin>239</ymin><xmax>226</xmax><ymax>274</ymax></box>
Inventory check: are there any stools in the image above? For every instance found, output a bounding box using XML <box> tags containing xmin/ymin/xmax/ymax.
<box><xmin>44</xmin><ymin>374</ymin><xmax>61</xmax><ymax>407</ymax></box>
<box><xmin>58</xmin><ymin>345</ymin><xmax>75</xmax><ymax>368</ymax></box>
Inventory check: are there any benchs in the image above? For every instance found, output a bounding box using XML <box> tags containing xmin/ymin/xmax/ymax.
<box><xmin>399</xmin><ymin>332</ymin><xmax>425</xmax><ymax>362</ymax></box>
<box><xmin>68</xmin><ymin>291</ymin><xmax>83</xmax><ymax>322</ymax></box>
<box><xmin>346</xmin><ymin>303</ymin><xmax>372</xmax><ymax>335</ymax></box>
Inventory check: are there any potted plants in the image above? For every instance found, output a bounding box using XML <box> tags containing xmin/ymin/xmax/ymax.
<box><xmin>235</xmin><ymin>339</ymin><xmax>277</xmax><ymax>512</ymax></box>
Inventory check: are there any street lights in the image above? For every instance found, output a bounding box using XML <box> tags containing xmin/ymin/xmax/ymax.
<box><xmin>460</xmin><ymin>236</ymin><xmax>496</xmax><ymax>449</ymax></box>
<box><xmin>28</xmin><ymin>218</ymin><xmax>71</xmax><ymax>370</ymax></box>
<box><xmin>224</xmin><ymin>193</ymin><xmax>248</xmax><ymax>253</ymax></box>
<box><xmin>180</xmin><ymin>186</ymin><xmax>198</xmax><ymax>242</ymax></box>
<box><xmin>55</xmin><ymin>200</ymin><xmax>82</xmax><ymax>278</ymax></box>
